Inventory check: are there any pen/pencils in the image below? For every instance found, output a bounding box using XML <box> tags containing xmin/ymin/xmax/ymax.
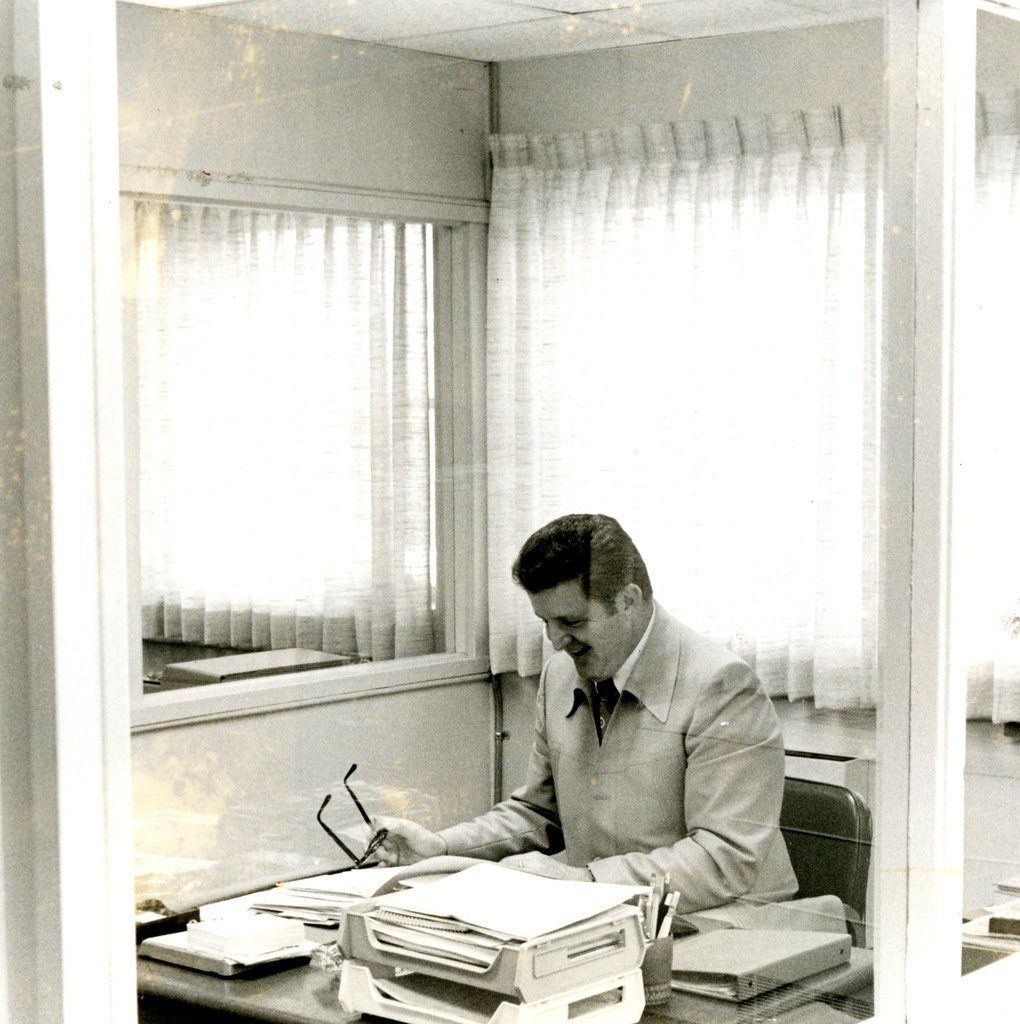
<box><xmin>637</xmin><ymin>873</ymin><xmax>681</xmax><ymax>940</ymax></box>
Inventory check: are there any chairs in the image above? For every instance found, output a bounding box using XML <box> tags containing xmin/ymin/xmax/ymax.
<box><xmin>780</xmin><ymin>772</ymin><xmax>876</xmax><ymax>953</ymax></box>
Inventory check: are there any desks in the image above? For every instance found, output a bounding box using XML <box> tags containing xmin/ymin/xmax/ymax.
<box><xmin>131</xmin><ymin>844</ymin><xmax>878</xmax><ymax>1024</ymax></box>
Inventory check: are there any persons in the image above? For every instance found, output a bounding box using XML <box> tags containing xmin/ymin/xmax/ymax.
<box><xmin>362</xmin><ymin>514</ymin><xmax>803</xmax><ymax>922</ymax></box>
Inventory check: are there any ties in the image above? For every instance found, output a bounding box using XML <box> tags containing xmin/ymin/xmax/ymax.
<box><xmin>596</xmin><ymin>678</ymin><xmax>620</xmax><ymax>745</ymax></box>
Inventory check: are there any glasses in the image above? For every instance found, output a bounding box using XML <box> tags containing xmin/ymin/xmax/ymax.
<box><xmin>317</xmin><ymin>763</ymin><xmax>389</xmax><ymax>865</ymax></box>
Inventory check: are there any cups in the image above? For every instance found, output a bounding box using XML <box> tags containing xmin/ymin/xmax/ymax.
<box><xmin>641</xmin><ymin>930</ymin><xmax>675</xmax><ymax>1007</ymax></box>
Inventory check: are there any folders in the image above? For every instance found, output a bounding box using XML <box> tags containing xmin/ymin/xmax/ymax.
<box><xmin>662</xmin><ymin>927</ymin><xmax>853</xmax><ymax>1003</ymax></box>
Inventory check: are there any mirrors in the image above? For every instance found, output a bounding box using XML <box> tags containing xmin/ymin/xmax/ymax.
<box><xmin>119</xmin><ymin>165</ymin><xmax>496</xmax><ymax>744</ymax></box>
